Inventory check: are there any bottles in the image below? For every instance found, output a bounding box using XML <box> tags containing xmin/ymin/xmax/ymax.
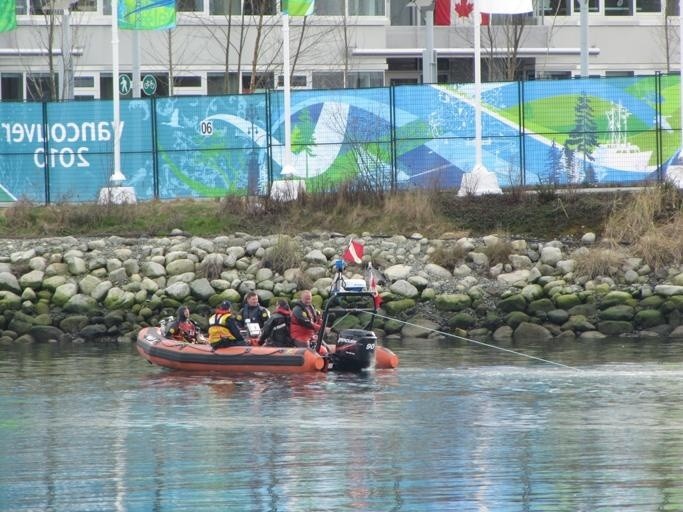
<box><xmin>222</xmin><ymin>301</ymin><xmax>231</xmax><ymax>309</ymax></box>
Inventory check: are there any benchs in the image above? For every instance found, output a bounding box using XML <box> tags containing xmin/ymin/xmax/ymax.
<box><xmin>116</xmin><ymin>1</ymin><xmax>176</xmax><ymax>30</ymax></box>
<box><xmin>282</xmin><ymin>0</ymin><xmax>315</xmax><ymax>16</ymax></box>
<box><xmin>0</xmin><ymin>1</ymin><xmax>17</xmax><ymax>33</ymax></box>
<box><xmin>344</xmin><ymin>240</ymin><xmax>364</xmax><ymax>265</ymax></box>
<box><xmin>479</xmin><ymin>0</ymin><xmax>533</xmax><ymax>15</ymax></box>
<box><xmin>370</xmin><ymin>275</ymin><xmax>380</xmax><ymax>311</ymax></box>
<box><xmin>432</xmin><ymin>0</ymin><xmax>491</xmax><ymax>28</ymax></box>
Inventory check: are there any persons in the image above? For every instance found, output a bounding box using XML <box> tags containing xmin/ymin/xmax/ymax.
<box><xmin>165</xmin><ymin>306</ymin><xmax>208</xmax><ymax>345</ymax></box>
<box><xmin>208</xmin><ymin>299</ymin><xmax>249</xmax><ymax>350</ymax></box>
<box><xmin>239</xmin><ymin>291</ymin><xmax>270</xmax><ymax>338</ymax></box>
<box><xmin>290</xmin><ymin>290</ymin><xmax>332</xmax><ymax>356</ymax></box>
<box><xmin>257</xmin><ymin>299</ymin><xmax>291</xmax><ymax>347</ymax></box>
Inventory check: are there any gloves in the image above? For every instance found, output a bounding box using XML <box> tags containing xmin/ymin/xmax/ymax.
<box><xmin>131</xmin><ymin>319</ymin><xmax>401</xmax><ymax>380</ymax></box>
